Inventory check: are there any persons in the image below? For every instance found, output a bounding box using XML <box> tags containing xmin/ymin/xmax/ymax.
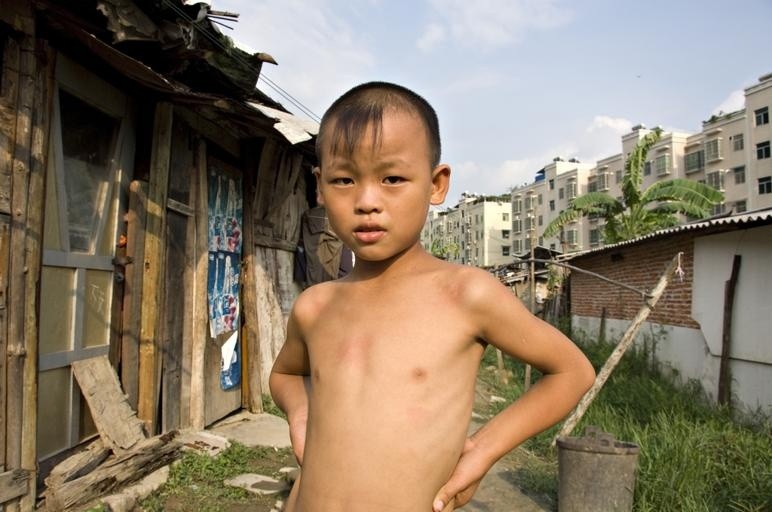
<box><xmin>210</xmin><ymin>215</ymin><xmax>242</xmax><ymax>253</ymax></box>
<box><xmin>266</xmin><ymin>78</ymin><xmax>597</xmax><ymax>512</ymax></box>
<box><xmin>209</xmin><ymin>293</ymin><xmax>237</xmax><ymax>333</ymax></box>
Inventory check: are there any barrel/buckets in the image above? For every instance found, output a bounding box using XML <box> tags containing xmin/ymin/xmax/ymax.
<box><xmin>556</xmin><ymin>425</ymin><xmax>640</xmax><ymax>512</ymax></box>
